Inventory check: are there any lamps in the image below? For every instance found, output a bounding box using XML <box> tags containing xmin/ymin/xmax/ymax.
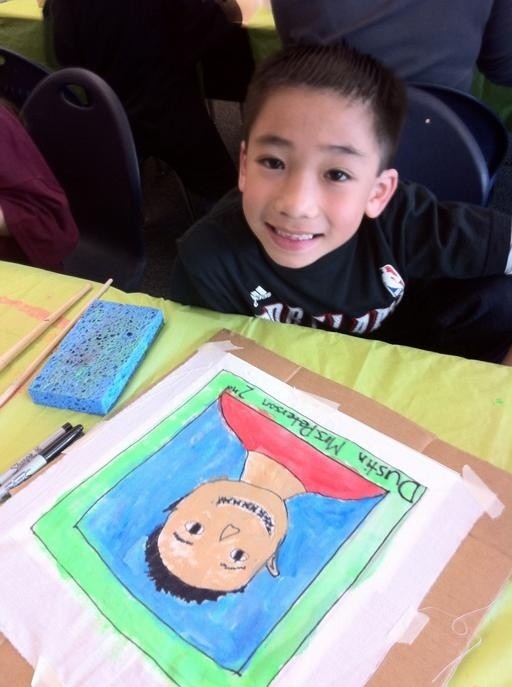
<box><xmin>382</xmin><ymin>83</ymin><xmax>512</xmax><ymax>365</ymax></box>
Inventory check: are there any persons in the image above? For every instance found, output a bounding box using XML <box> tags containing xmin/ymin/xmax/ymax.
<box><xmin>0</xmin><ymin>99</ymin><xmax>82</xmax><ymax>271</ymax></box>
<box><xmin>165</xmin><ymin>39</ymin><xmax>512</xmax><ymax>336</ymax></box>
<box><xmin>143</xmin><ymin>391</ymin><xmax>386</xmax><ymax>607</ymax></box>
<box><xmin>45</xmin><ymin>0</ymin><xmax>242</xmax><ymax>192</ymax></box>
<box><xmin>272</xmin><ymin>0</ymin><xmax>512</xmax><ymax>105</ymax></box>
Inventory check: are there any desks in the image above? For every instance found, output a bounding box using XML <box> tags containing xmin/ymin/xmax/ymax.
<box><xmin>0</xmin><ymin>262</ymin><xmax>508</xmax><ymax>684</ymax></box>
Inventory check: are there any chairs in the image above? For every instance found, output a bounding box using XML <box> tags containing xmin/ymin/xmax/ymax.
<box><xmin>0</xmin><ymin>44</ymin><xmax>199</xmax><ymax>294</ymax></box>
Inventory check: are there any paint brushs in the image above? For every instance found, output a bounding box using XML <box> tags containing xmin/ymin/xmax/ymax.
<box><xmin>0</xmin><ymin>278</ymin><xmax>116</xmax><ymax>417</ymax></box>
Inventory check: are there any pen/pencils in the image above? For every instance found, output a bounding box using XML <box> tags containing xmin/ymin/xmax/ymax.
<box><xmin>3</xmin><ymin>421</ymin><xmax>84</xmax><ymax>498</ymax></box>
